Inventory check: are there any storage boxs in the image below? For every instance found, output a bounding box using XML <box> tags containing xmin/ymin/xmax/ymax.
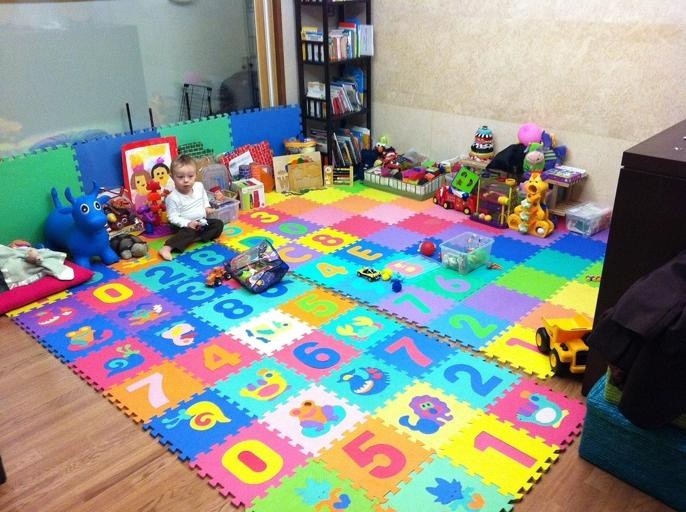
<box><xmin>206</xmin><ymin>191</ymin><xmax>240</xmax><ymax>225</ymax></box>
<box><xmin>439</xmin><ymin>232</ymin><xmax>494</xmax><ymax>273</ymax></box>
<box><xmin>566</xmin><ymin>200</ymin><xmax>614</xmax><ymax>235</ymax></box>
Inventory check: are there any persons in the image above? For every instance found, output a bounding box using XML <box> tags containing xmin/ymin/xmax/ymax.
<box><xmin>159</xmin><ymin>155</ymin><xmax>223</xmax><ymax>261</ymax></box>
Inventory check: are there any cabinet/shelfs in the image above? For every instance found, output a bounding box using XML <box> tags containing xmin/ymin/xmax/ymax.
<box><xmin>295</xmin><ymin>0</ymin><xmax>372</xmax><ymax>166</ymax></box>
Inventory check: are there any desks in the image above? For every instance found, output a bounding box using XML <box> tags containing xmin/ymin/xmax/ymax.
<box><xmin>581</xmin><ymin>120</ymin><xmax>686</xmax><ymax>397</ymax></box>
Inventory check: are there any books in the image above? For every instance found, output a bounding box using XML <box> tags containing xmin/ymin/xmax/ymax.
<box><xmin>300</xmin><ymin>69</ymin><xmax>366</xmax><ymax>119</ymax></box>
<box><xmin>301</xmin><ymin>16</ymin><xmax>375</xmax><ymax>61</ymax></box>
<box><xmin>305</xmin><ymin>127</ymin><xmax>370</xmax><ymax>166</ymax></box>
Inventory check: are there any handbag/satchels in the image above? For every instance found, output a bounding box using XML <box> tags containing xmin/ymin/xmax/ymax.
<box><xmin>228</xmin><ymin>239</ymin><xmax>290</xmax><ymax>295</ymax></box>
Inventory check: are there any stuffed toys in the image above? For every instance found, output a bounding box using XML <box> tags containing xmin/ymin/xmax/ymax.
<box><xmin>112</xmin><ymin>233</ymin><xmax>148</xmax><ymax>259</ymax></box>
<box><xmin>356</xmin><ymin>143</ymin><xmax>385</xmax><ymax>180</ymax></box>
